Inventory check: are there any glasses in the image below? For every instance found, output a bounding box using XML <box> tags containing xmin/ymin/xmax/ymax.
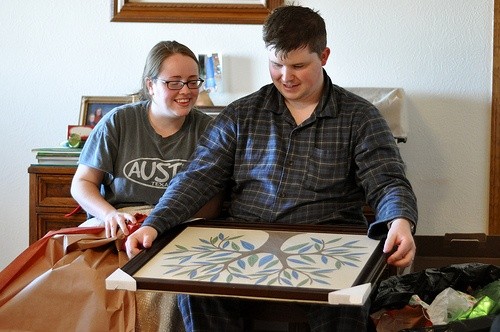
<box><xmin>153</xmin><ymin>76</ymin><xmax>204</xmax><ymax>90</ymax></box>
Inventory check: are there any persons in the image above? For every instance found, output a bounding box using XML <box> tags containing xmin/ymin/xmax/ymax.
<box><xmin>90</xmin><ymin>108</ymin><xmax>102</xmax><ymax>127</ymax></box>
<box><xmin>72</xmin><ymin>41</ymin><xmax>223</xmax><ymax>237</ymax></box>
<box><xmin>125</xmin><ymin>6</ymin><xmax>418</xmax><ymax>332</ymax></box>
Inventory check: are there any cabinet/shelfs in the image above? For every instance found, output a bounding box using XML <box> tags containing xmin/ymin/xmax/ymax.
<box><xmin>27</xmin><ymin>166</ymin><xmax>87</xmax><ymax>246</ymax></box>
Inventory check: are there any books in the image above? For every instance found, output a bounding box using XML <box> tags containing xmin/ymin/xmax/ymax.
<box><xmin>32</xmin><ymin>147</ymin><xmax>82</xmax><ymax>166</ymax></box>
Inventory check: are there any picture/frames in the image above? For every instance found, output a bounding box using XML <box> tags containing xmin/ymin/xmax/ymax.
<box><xmin>110</xmin><ymin>0</ymin><xmax>284</xmax><ymax>24</ymax></box>
<box><xmin>79</xmin><ymin>96</ymin><xmax>134</xmax><ymax>128</ymax></box>
<box><xmin>105</xmin><ymin>216</ymin><xmax>397</xmax><ymax>306</ymax></box>
